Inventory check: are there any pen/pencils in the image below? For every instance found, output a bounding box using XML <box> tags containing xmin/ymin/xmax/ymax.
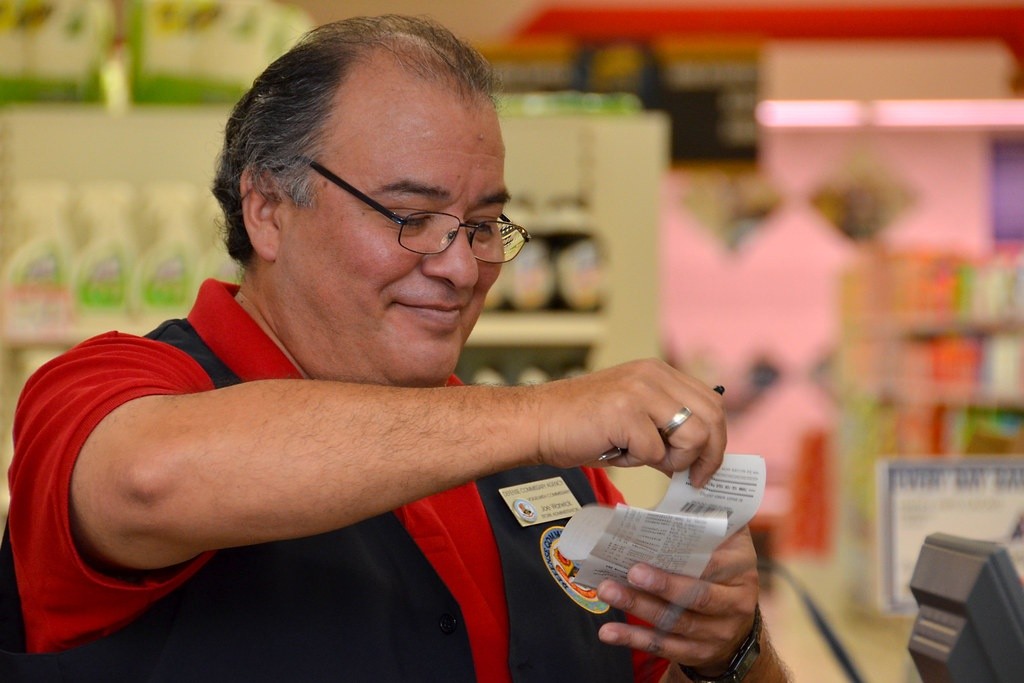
<box><xmin>597</xmin><ymin>384</ymin><xmax>725</xmax><ymax>462</ymax></box>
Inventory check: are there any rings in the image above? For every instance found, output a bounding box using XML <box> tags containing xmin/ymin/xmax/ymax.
<box><xmin>661</xmin><ymin>405</ymin><xmax>691</xmax><ymax>438</ymax></box>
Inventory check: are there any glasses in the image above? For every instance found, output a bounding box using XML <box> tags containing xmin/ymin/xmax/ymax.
<box><xmin>295</xmin><ymin>155</ymin><xmax>530</xmax><ymax>262</ymax></box>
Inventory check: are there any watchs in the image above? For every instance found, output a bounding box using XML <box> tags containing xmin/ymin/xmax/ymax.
<box><xmin>678</xmin><ymin>601</ymin><xmax>762</xmax><ymax>682</ymax></box>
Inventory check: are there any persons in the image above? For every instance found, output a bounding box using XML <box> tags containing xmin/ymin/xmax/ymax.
<box><xmin>7</xmin><ymin>10</ymin><xmax>797</xmax><ymax>682</ymax></box>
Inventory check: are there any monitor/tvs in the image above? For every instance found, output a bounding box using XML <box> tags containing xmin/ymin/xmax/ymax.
<box><xmin>906</xmin><ymin>531</ymin><xmax>1024</xmax><ymax>683</ymax></box>
